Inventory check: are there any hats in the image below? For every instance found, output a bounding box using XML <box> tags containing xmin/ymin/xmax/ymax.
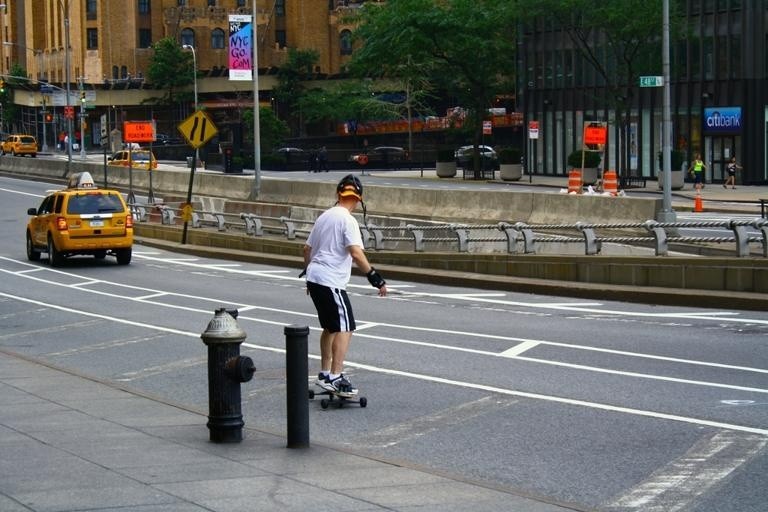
<box><xmin>336</xmin><ymin>174</ymin><xmax>362</xmax><ymax>200</ymax></box>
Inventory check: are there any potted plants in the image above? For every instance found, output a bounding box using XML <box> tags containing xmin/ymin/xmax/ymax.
<box><xmin>436</xmin><ymin>146</ymin><xmax>457</xmax><ymax>178</ymax></box>
<box><xmin>657</xmin><ymin>150</ymin><xmax>686</xmax><ymax>189</ymax></box>
<box><xmin>499</xmin><ymin>148</ymin><xmax>522</xmax><ymax>180</ymax></box>
<box><xmin>569</xmin><ymin>150</ymin><xmax>602</xmax><ymax>185</ymax></box>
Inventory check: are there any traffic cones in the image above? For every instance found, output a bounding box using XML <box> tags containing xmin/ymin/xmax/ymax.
<box><xmin>693</xmin><ymin>183</ymin><xmax>703</xmax><ymax>212</ymax></box>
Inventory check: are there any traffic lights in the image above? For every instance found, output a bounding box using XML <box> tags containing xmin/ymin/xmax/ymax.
<box><xmin>79</xmin><ymin>91</ymin><xmax>86</xmax><ymax>103</ymax></box>
<box><xmin>0</xmin><ymin>79</ymin><xmax>4</xmax><ymax>92</ymax></box>
<box><xmin>45</xmin><ymin>113</ymin><xmax>53</xmax><ymax>122</ymax></box>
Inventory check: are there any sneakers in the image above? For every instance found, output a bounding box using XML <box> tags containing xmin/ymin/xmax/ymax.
<box><xmin>314</xmin><ymin>372</ymin><xmax>359</xmax><ymax>397</ymax></box>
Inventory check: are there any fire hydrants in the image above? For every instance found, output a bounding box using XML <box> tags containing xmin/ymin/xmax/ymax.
<box><xmin>201</xmin><ymin>307</ymin><xmax>256</xmax><ymax>444</ymax></box>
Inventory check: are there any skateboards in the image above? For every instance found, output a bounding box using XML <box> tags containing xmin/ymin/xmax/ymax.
<box><xmin>309</xmin><ymin>377</ymin><xmax>366</xmax><ymax>410</ymax></box>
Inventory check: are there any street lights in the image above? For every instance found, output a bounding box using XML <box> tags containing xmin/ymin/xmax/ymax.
<box><xmin>182</xmin><ymin>44</ymin><xmax>201</xmax><ymax>167</ymax></box>
<box><xmin>2</xmin><ymin>41</ymin><xmax>49</xmax><ymax>152</ymax></box>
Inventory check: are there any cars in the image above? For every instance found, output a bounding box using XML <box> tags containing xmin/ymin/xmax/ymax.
<box><xmin>0</xmin><ymin>135</ymin><xmax>38</xmax><ymax>157</ymax></box>
<box><xmin>277</xmin><ymin>147</ymin><xmax>304</xmax><ymax>152</ymax></box>
<box><xmin>27</xmin><ymin>172</ymin><xmax>135</xmax><ymax>266</ymax></box>
<box><xmin>108</xmin><ymin>143</ymin><xmax>157</xmax><ymax>170</ymax></box>
<box><xmin>454</xmin><ymin>145</ymin><xmax>497</xmax><ymax>163</ymax></box>
<box><xmin>347</xmin><ymin>146</ymin><xmax>408</xmax><ymax>163</ymax></box>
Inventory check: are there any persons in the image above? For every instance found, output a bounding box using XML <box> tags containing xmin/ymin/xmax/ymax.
<box><xmin>722</xmin><ymin>156</ymin><xmax>743</xmax><ymax>190</ymax></box>
<box><xmin>312</xmin><ymin>145</ymin><xmax>321</xmax><ymax>172</ymax></box>
<box><xmin>58</xmin><ymin>130</ymin><xmax>67</xmax><ymax>150</ymax></box>
<box><xmin>303</xmin><ymin>175</ymin><xmax>388</xmax><ymax>394</ymax></box>
<box><xmin>687</xmin><ymin>153</ymin><xmax>707</xmax><ymax>189</ymax></box>
<box><xmin>319</xmin><ymin>146</ymin><xmax>329</xmax><ymax>172</ymax></box>
<box><xmin>64</xmin><ymin>135</ymin><xmax>68</xmax><ymax>154</ymax></box>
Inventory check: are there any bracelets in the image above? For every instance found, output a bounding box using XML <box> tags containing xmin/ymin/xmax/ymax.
<box><xmin>365</xmin><ymin>265</ymin><xmax>386</xmax><ymax>288</ymax></box>
